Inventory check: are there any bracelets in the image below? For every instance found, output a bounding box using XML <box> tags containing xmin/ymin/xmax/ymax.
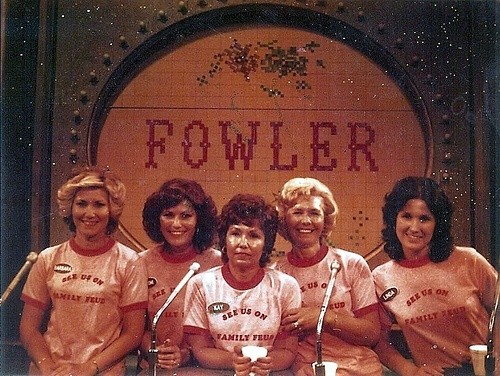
<box><xmin>34</xmin><ymin>355</ymin><xmax>50</xmax><ymax>365</ymax></box>
<box><xmin>91</xmin><ymin>358</ymin><xmax>99</xmax><ymax>375</ymax></box>
<box><xmin>330</xmin><ymin>311</ymin><xmax>337</xmax><ymax>335</ymax></box>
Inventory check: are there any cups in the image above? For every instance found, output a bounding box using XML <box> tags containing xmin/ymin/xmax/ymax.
<box><xmin>469</xmin><ymin>345</ymin><xmax>488</xmax><ymax>376</ymax></box>
<box><xmin>242</xmin><ymin>345</ymin><xmax>268</xmax><ymax>376</ymax></box>
<box><xmin>312</xmin><ymin>361</ymin><xmax>337</xmax><ymax>376</ymax></box>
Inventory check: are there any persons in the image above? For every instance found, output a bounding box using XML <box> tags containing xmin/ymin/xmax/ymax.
<box><xmin>183</xmin><ymin>194</ymin><xmax>302</xmax><ymax>376</ymax></box>
<box><xmin>19</xmin><ymin>166</ymin><xmax>149</xmax><ymax>376</ymax></box>
<box><xmin>264</xmin><ymin>178</ymin><xmax>383</xmax><ymax>376</ymax></box>
<box><xmin>137</xmin><ymin>178</ymin><xmax>223</xmax><ymax>369</ymax></box>
<box><xmin>372</xmin><ymin>176</ymin><xmax>500</xmax><ymax>376</ymax></box>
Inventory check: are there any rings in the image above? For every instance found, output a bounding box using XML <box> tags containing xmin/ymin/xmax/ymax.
<box><xmin>292</xmin><ymin>321</ymin><xmax>302</xmax><ymax>334</ymax></box>
<box><xmin>266</xmin><ymin>370</ymin><xmax>269</xmax><ymax>376</ymax></box>
<box><xmin>68</xmin><ymin>373</ymin><xmax>73</xmax><ymax>376</ymax></box>
<box><xmin>170</xmin><ymin>359</ymin><xmax>173</xmax><ymax>366</ymax></box>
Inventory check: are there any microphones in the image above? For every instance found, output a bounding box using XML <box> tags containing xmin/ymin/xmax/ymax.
<box><xmin>149</xmin><ymin>262</ymin><xmax>200</xmax><ymax>376</ymax></box>
<box><xmin>315</xmin><ymin>259</ymin><xmax>341</xmax><ymax>376</ymax></box>
<box><xmin>0</xmin><ymin>252</ymin><xmax>37</xmax><ymax>306</ymax></box>
